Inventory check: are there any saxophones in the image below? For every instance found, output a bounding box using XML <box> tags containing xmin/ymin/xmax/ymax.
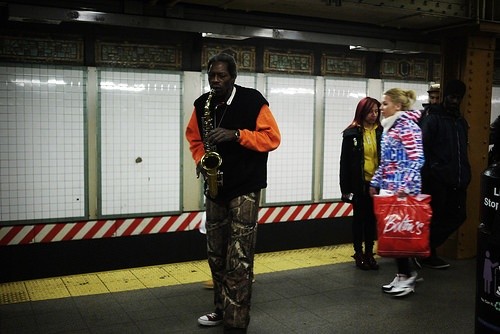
<box><xmin>201</xmin><ymin>89</ymin><xmax>223</xmax><ymax>203</ymax></box>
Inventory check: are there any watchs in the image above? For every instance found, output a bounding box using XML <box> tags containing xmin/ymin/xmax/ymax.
<box><xmin>235</xmin><ymin>131</ymin><xmax>240</xmax><ymax>138</ymax></box>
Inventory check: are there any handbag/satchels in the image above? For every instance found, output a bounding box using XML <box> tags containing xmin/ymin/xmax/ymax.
<box><xmin>372</xmin><ymin>188</ymin><xmax>432</xmax><ymax>258</ymax></box>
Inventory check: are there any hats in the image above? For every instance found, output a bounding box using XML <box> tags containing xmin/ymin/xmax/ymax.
<box><xmin>427</xmin><ymin>88</ymin><xmax>440</xmax><ymax>93</ymax></box>
<box><xmin>443</xmin><ymin>79</ymin><xmax>466</xmax><ymax>97</ymax></box>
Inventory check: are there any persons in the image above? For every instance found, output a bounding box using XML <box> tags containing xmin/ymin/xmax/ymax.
<box><xmin>186</xmin><ymin>53</ymin><xmax>281</xmax><ymax>334</ymax></box>
<box><xmin>369</xmin><ymin>88</ymin><xmax>425</xmax><ymax>297</ymax></box>
<box><xmin>339</xmin><ymin>97</ymin><xmax>384</xmax><ymax>270</ymax></box>
<box><xmin>418</xmin><ymin>80</ymin><xmax>472</xmax><ymax>268</ymax></box>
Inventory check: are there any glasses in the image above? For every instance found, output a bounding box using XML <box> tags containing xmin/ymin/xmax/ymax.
<box><xmin>430</xmin><ymin>96</ymin><xmax>439</xmax><ymax>100</ymax></box>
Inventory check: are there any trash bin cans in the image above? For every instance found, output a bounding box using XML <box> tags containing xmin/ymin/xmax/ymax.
<box><xmin>475</xmin><ymin>164</ymin><xmax>500</xmax><ymax>334</ymax></box>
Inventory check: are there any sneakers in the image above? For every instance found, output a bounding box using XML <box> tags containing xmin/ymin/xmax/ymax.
<box><xmin>198</xmin><ymin>312</ymin><xmax>224</xmax><ymax>326</ymax></box>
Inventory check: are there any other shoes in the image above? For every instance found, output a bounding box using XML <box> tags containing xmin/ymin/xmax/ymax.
<box><xmin>363</xmin><ymin>252</ymin><xmax>379</xmax><ymax>267</ymax></box>
<box><xmin>414</xmin><ymin>255</ymin><xmax>451</xmax><ymax>269</ymax></box>
<box><xmin>353</xmin><ymin>253</ymin><xmax>366</xmax><ymax>270</ymax></box>
<box><xmin>381</xmin><ymin>274</ymin><xmax>417</xmax><ymax>297</ymax></box>
